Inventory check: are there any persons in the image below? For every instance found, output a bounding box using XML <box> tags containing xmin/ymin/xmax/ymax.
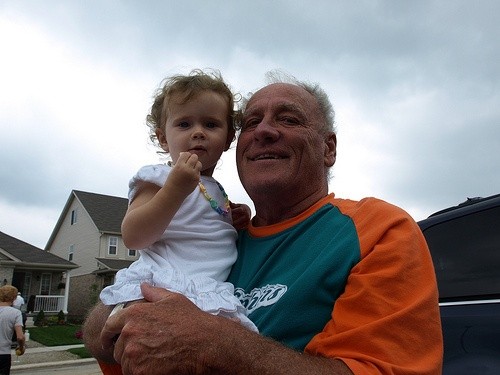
<box><xmin>84</xmin><ymin>69</ymin><xmax>443</xmax><ymax>375</ymax></box>
<box><xmin>0</xmin><ymin>285</ymin><xmax>25</xmax><ymax>375</ymax></box>
<box><xmin>99</xmin><ymin>69</ymin><xmax>260</xmax><ymax>334</ymax></box>
<box><xmin>13</xmin><ymin>293</ymin><xmax>25</xmax><ymax>311</ymax></box>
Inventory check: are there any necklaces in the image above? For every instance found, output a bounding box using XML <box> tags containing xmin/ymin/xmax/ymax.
<box><xmin>167</xmin><ymin>161</ymin><xmax>230</xmax><ymax>216</ymax></box>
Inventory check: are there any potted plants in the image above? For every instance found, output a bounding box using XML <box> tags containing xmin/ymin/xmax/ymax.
<box><xmin>57</xmin><ymin>310</ymin><xmax>65</xmax><ymax>325</ymax></box>
<box><xmin>34</xmin><ymin>310</ymin><xmax>49</xmax><ymax>327</ymax></box>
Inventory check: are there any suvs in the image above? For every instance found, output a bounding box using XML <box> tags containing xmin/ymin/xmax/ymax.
<box><xmin>415</xmin><ymin>193</ymin><xmax>500</xmax><ymax>375</ymax></box>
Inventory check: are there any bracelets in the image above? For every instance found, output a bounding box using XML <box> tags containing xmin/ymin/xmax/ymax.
<box><xmin>108</xmin><ymin>302</ymin><xmax>127</xmax><ymax>317</ymax></box>
